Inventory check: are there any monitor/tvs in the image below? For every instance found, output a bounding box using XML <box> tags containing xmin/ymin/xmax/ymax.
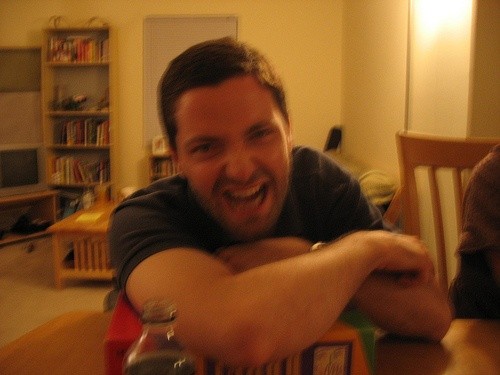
<box><xmin>0</xmin><ymin>142</ymin><xmax>49</xmax><ymax>198</ymax></box>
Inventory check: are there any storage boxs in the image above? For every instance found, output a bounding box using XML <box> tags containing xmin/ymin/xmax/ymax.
<box><xmin>104</xmin><ymin>290</ymin><xmax>377</xmax><ymax>375</ymax></box>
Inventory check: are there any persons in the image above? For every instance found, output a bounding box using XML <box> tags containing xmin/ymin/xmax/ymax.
<box><xmin>107</xmin><ymin>37</ymin><xmax>453</xmax><ymax>371</ymax></box>
<box><xmin>445</xmin><ymin>145</ymin><xmax>500</xmax><ymax>319</ymax></box>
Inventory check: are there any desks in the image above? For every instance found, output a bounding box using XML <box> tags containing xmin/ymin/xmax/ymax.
<box><xmin>0</xmin><ymin>189</ymin><xmax>60</xmax><ymax>249</ymax></box>
<box><xmin>46</xmin><ymin>200</ymin><xmax>122</xmax><ymax>290</ymax></box>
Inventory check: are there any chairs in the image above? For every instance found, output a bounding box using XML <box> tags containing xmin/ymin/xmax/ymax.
<box><xmin>395</xmin><ymin>131</ymin><xmax>500</xmax><ymax>298</ymax></box>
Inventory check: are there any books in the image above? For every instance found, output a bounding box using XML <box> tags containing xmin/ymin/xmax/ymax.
<box><xmin>49</xmin><ymin>36</ymin><xmax>108</xmax><ymax>63</ymax></box>
<box><xmin>51</xmin><ymin>154</ymin><xmax>110</xmax><ymax>185</ymax></box>
<box><xmin>72</xmin><ymin>238</ymin><xmax>112</xmax><ymax>272</ymax></box>
<box><xmin>53</xmin><ymin>119</ymin><xmax>110</xmax><ymax>146</ymax></box>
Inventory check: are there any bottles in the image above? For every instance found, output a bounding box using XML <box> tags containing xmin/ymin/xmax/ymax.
<box><xmin>122</xmin><ymin>298</ymin><xmax>195</xmax><ymax>375</ymax></box>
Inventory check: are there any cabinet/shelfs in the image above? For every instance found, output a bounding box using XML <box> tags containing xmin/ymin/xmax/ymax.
<box><xmin>42</xmin><ymin>24</ymin><xmax>133</xmax><ymax>222</ymax></box>
